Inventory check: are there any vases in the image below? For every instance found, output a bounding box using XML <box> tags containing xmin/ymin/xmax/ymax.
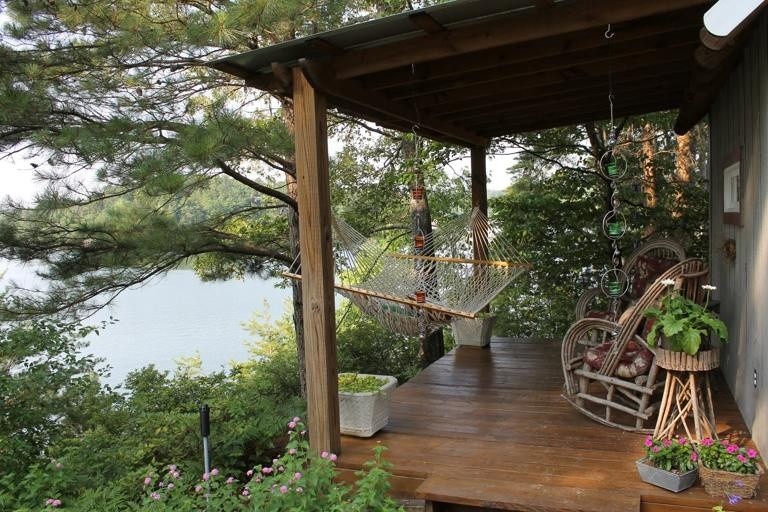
<box><xmin>634</xmin><ymin>456</ymin><xmax>766</xmax><ymax>500</ymax></box>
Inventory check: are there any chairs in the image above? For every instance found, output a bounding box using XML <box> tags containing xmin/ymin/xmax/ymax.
<box><xmin>560</xmin><ymin>240</ymin><xmax>710</xmax><ymax>435</ymax></box>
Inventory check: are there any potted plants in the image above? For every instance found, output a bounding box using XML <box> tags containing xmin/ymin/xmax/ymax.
<box><xmin>451</xmin><ymin>313</ymin><xmax>497</xmax><ymax>349</ymax></box>
<box><xmin>337</xmin><ymin>373</ymin><xmax>398</xmax><ymax>438</ymax></box>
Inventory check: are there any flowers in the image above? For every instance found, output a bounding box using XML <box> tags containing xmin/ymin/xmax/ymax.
<box><xmin>642</xmin><ymin>432</ymin><xmax>761</xmax><ymax>475</ymax></box>
<box><xmin>639</xmin><ymin>278</ymin><xmax>729</xmax><ymax>355</ymax></box>
